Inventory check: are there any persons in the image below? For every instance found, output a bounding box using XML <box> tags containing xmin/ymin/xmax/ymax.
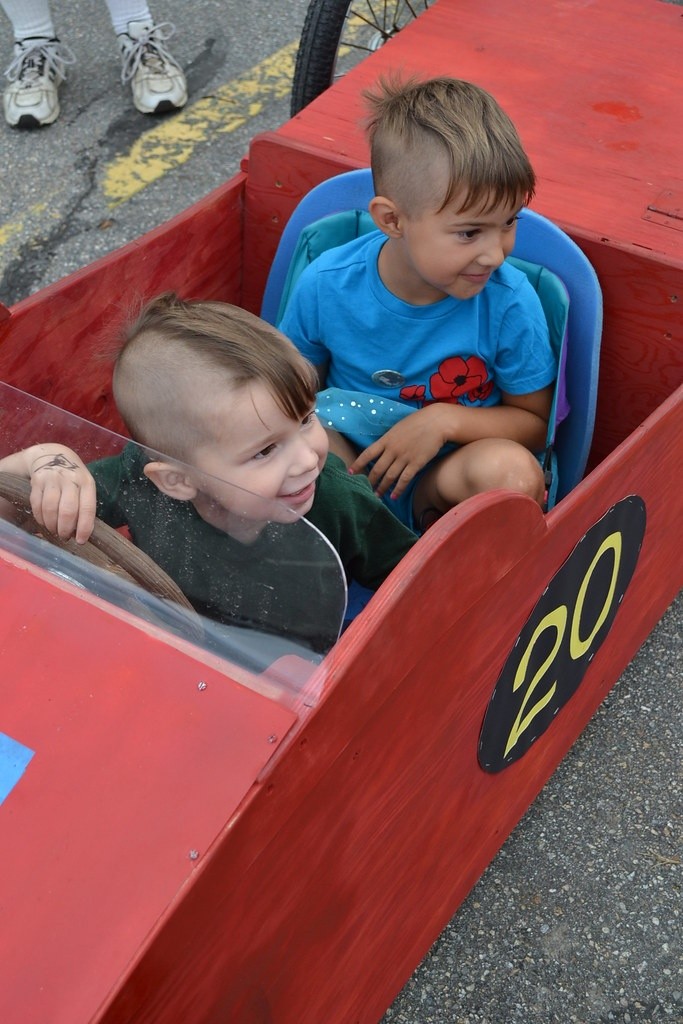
<box><xmin>0</xmin><ymin>0</ymin><xmax>187</xmax><ymax>129</ymax></box>
<box><xmin>0</xmin><ymin>288</ymin><xmax>417</xmax><ymax>654</ymax></box>
<box><xmin>276</xmin><ymin>76</ymin><xmax>556</xmax><ymax>532</ymax></box>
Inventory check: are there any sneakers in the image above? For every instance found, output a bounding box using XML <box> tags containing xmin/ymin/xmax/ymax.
<box><xmin>3</xmin><ymin>35</ymin><xmax>79</xmax><ymax>129</ymax></box>
<box><xmin>117</xmin><ymin>19</ymin><xmax>188</xmax><ymax>112</ymax></box>
